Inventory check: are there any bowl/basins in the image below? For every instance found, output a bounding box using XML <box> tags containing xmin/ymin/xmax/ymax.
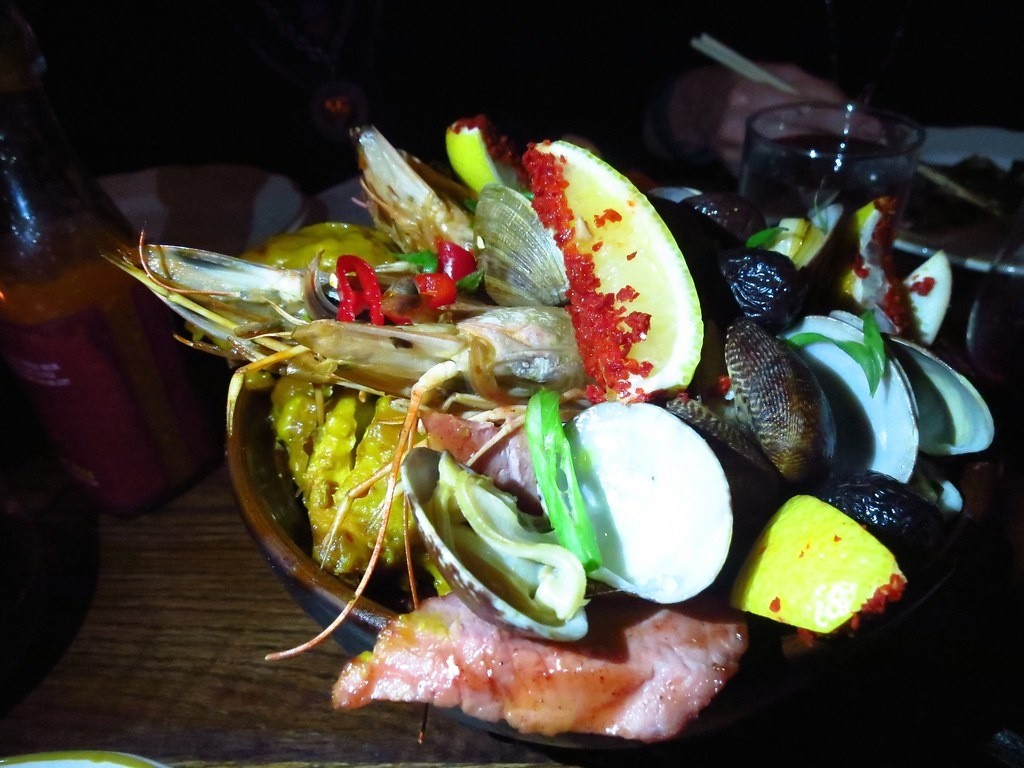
<box><xmin>226</xmin><ymin>344</ymin><xmax>836</xmax><ymax>741</ymax></box>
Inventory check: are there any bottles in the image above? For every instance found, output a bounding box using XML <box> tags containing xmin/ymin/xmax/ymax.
<box><xmin>0</xmin><ymin>0</ymin><xmax>217</xmax><ymax>519</ymax></box>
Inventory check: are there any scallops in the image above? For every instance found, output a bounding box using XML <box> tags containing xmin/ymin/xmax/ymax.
<box><xmin>666</xmin><ymin>315</ymin><xmax>996</xmax><ymax>516</ymax></box>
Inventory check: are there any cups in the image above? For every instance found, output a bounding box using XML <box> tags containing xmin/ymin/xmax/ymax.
<box><xmin>738</xmin><ymin>101</ymin><xmax>927</xmax><ymax>285</ymax></box>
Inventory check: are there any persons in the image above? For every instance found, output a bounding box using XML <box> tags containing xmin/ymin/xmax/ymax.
<box><xmin>584</xmin><ymin>0</ymin><xmax>1023</xmax><ymax>201</ymax></box>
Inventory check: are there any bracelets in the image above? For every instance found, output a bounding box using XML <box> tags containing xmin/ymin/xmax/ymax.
<box><xmin>649</xmin><ymin>76</ymin><xmax>713</xmax><ymax>169</ymax></box>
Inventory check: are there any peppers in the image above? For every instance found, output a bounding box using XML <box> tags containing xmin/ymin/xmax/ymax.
<box><xmin>333</xmin><ymin>241</ymin><xmax>476</xmax><ymax>325</ymax></box>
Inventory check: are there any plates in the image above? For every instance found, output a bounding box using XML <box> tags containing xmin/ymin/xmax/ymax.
<box><xmin>96</xmin><ymin>166</ymin><xmax>308</xmax><ymax>257</ymax></box>
<box><xmin>892</xmin><ymin>127</ymin><xmax>1024</xmax><ymax>276</ymax></box>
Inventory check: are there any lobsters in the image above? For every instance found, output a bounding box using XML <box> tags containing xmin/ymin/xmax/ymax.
<box><xmin>109</xmin><ymin>109</ymin><xmax>598</xmax><ymax>666</ymax></box>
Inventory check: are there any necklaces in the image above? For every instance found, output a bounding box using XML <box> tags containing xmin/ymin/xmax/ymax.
<box><xmin>221</xmin><ymin>0</ymin><xmax>384</xmax><ymax>142</ymax></box>
<box><xmin>825</xmin><ymin>0</ymin><xmax>912</xmax><ymax>101</ymax></box>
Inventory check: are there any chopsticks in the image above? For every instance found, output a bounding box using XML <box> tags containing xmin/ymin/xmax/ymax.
<box><xmin>692</xmin><ymin>34</ymin><xmax>982</xmax><ymax>208</ymax></box>
